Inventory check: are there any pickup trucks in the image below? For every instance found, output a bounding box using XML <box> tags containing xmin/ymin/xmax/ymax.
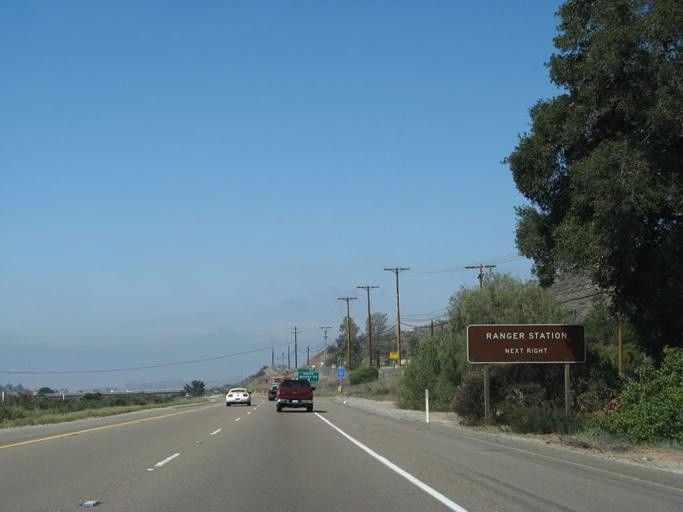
<box><xmin>275</xmin><ymin>377</ymin><xmax>316</xmax><ymax>413</ymax></box>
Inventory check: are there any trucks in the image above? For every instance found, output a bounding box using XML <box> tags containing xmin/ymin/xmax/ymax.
<box><xmin>263</xmin><ymin>376</ymin><xmax>291</xmax><ymax>402</ymax></box>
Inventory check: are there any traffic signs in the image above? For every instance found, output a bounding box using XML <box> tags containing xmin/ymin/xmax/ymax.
<box><xmin>465</xmin><ymin>324</ymin><xmax>586</xmax><ymax>363</ymax></box>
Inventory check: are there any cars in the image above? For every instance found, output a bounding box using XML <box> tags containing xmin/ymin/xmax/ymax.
<box><xmin>185</xmin><ymin>392</ymin><xmax>192</xmax><ymax>397</ymax></box>
<box><xmin>225</xmin><ymin>388</ymin><xmax>251</xmax><ymax>406</ymax></box>
